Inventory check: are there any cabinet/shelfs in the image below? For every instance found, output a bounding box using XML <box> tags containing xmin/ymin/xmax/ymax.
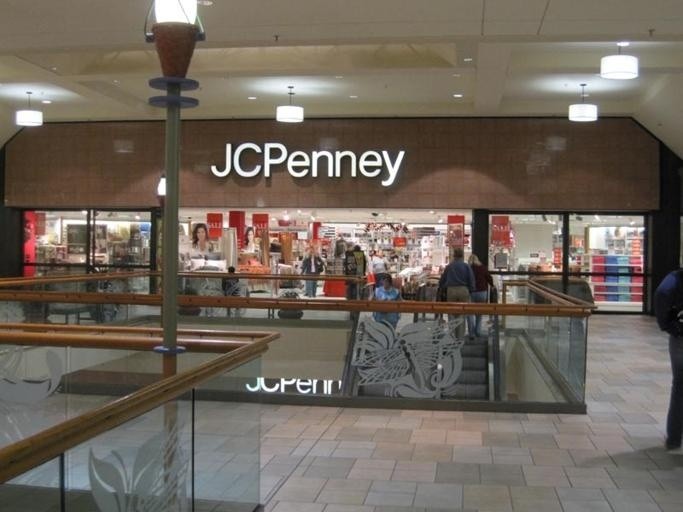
<box><xmin>37</xmin><ymin>242</ymin><xmax>68</xmax><ymax>271</ymax></box>
<box><xmin>580</xmin><ymin>250</ymin><xmax>644</xmax><ymax>306</ymax></box>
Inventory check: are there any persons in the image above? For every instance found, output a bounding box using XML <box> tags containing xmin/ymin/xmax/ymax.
<box><xmin>222</xmin><ymin>267</ymin><xmax>239</xmax><ymax>316</ymax></box>
<box><xmin>192</xmin><ymin>224</ymin><xmax>209</xmax><ymax>252</ymax></box>
<box><xmin>440</xmin><ymin>248</ymin><xmax>475</xmax><ymax>338</ymax></box>
<box><xmin>301</xmin><ymin>245</ymin><xmax>390</xmax><ymax>300</ymax></box>
<box><xmin>655</xmin><ymin>268</ymin><xmax>683</xmax><ymax>453</ymax></box>
<box><xmin>368</xmin><ymin>274</ymin><xmax>403</xmax><ymax>336</ymax></box>
<box><xmin>467</xmin><ymin>254</ymin><xmax>494</xmax><ymax>339</ymax></box>
<box><xmin>244</xmin><ymin>227</ymin><xmax>254</xmax><ymax>252</ymax></box>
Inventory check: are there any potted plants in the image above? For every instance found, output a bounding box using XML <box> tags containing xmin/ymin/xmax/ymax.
<box><xmin>274</xmin><ymin>290</ymin><xmax>306</xmax><ymax>320</ymax></box>
<box><xmin>13</xmin><ymin>280</ymin><xmax>48</xmax><ymax>326</ymax></box>
<box><xmin>175</xmin><ymin>286</ymin><xmax>203</xmax><ymax>316</ymax></box>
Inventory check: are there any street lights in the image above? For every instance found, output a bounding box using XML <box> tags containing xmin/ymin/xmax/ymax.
<box><xmin>143</xmin><ymin>1</ymin><xmax>204</xmax><ymax>510</ymax></box>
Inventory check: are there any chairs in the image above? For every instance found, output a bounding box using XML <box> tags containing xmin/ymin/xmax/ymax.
<box><xmin>199</xmin><ymin>276</ymin><xmax>224</xmax><ymax>317</ymax></box>
<box><xmin>226</xmin><ymin>280</ymin><xmax>254</xmax><ymax>317</ymax></box>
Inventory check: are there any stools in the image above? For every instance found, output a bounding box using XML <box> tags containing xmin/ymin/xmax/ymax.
<box><xmin>50</xmin><ymin>299</ymin><xmax>101</xmax><ymax>325</ymax></box>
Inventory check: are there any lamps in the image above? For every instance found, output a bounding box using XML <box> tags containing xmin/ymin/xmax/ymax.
<box><xmin>566</xmin><ymin>82</ymin><xmax>598</xmax><ymax>123</ymax></box>
<box><xmin>15</xmin><ymin>90</ymin><xmax>43</xmax><ymax>127</ymax></box>
<box><xmin>274</xmin><ymin>83</ymin><xmax>304</xmax><ymax>125</ymax></box>
<box><xmin>599</xmin><ymin>42</ymin><xmax>640</xmax><ymax>82</ymax></box>
